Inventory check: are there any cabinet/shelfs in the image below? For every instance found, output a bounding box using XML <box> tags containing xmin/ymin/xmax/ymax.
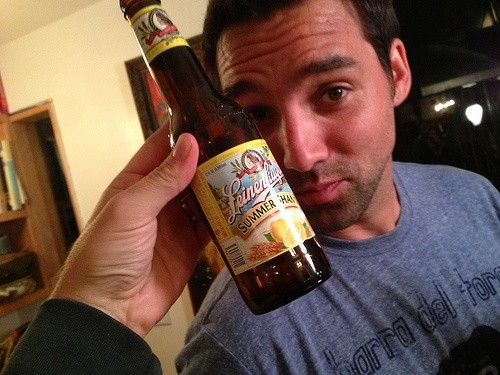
<box><xmin>0</xmin><ymin>157</ymin><xmax>52</xmax><ymax>371</ymax></box>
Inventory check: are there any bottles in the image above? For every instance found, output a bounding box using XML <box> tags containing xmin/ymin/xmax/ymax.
<box><xmin>119</xmin><ymin>0</ymin><xmax>333</xmax><ymax>314</ymax></box>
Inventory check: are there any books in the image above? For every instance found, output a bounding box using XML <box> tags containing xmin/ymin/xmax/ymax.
<box><xmin>0</xmin><ymin>139</ymin><xmax>27</xmax><ymax>214</ymax></box>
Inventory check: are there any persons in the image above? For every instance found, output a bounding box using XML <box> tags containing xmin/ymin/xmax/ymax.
<box><xmin>0</xmin><ymin>117</ymin><xmax>212</xmax><ymax>375</ymax></box>
<box><xmin>175</xmin><ymin>0</ymin><xmax>500</xmax><ymax>375</ymax></box>
